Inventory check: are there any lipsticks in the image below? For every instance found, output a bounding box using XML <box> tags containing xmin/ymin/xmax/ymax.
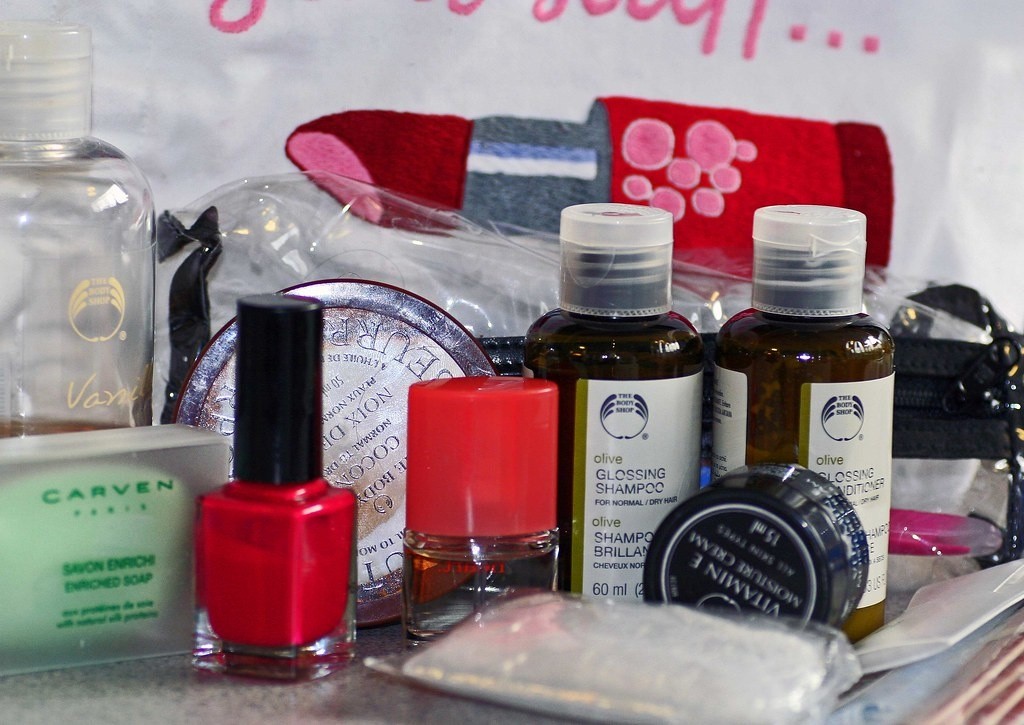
<box><xmin>284</xmin><ymin>97</ymin><xmax>892</xmax><ymax>269</ymax></box>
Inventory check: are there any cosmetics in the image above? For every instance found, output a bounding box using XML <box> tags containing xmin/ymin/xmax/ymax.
<box><xmin>0</xmin><ymin>22</ymin><xmax>894</xmax><ymax>684</ymax></box>
